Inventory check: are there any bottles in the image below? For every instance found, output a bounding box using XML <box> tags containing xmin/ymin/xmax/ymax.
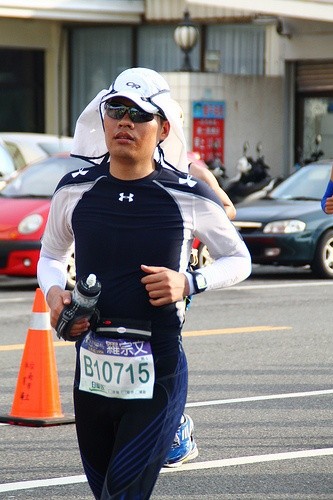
<box><xmin>70</xmin><ymin>274</ymin><xmax>101</xmax><ymax>320</ymax></box>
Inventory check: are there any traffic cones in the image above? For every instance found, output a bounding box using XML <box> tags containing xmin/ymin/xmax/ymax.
<box><xmin>0</xmin><ymin>287</ymin><xmax>75</xmax><ymax>427</ymax></box>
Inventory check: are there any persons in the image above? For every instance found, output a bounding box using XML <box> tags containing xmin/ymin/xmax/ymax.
<box><xmin>321</xmin><ymin>168</ymin><xmax>333</xmax><ymax>214</ymax></box>
<box><xmin>39</xmin><ymin>68</ymin><xmax>253</xmax><ymax>500</ymax></box>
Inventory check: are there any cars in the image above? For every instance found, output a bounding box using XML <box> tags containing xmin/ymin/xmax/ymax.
<box><xmin>229</xmin><ymin>158</ymin><xmax>333</xmax><ymax>282</ymax></box>
<box><xmin>0</xmin><ymin>131</ymin><xmax>214</xmax><ymax>292</ymax></box>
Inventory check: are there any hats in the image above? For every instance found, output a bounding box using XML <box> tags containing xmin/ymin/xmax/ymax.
<box><xmin>101</xmin><ymin>67</ymin><xmax>171</xmax><ymax>113</ymax></box>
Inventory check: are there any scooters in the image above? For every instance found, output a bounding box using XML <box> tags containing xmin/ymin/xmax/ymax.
<box><xmin>206</xmin><ymin>134</ymin><xmax>325</xmax><ymax>210</ymax></box>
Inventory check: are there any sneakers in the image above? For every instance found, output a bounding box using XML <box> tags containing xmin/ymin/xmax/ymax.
<box><xmin>163</xmin><ymin>413</ymin><xmax>194</xmax><ymax>468</ymax></box>
<box><xmin>182</xmin><ymin>435</ymin><xmax>199</xmax><ymax>462</ymax></box>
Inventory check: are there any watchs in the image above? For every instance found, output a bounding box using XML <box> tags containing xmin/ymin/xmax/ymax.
<box><xmin>187</xmin><ymin>270</ymin><xmax>208</xmax><ymax>296</ymax></box>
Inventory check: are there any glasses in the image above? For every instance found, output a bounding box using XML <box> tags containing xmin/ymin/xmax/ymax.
<box><xmin>103</xmin><ymin>101</ymin><xmax>154</xmax><ymax>125</ymax></box>
